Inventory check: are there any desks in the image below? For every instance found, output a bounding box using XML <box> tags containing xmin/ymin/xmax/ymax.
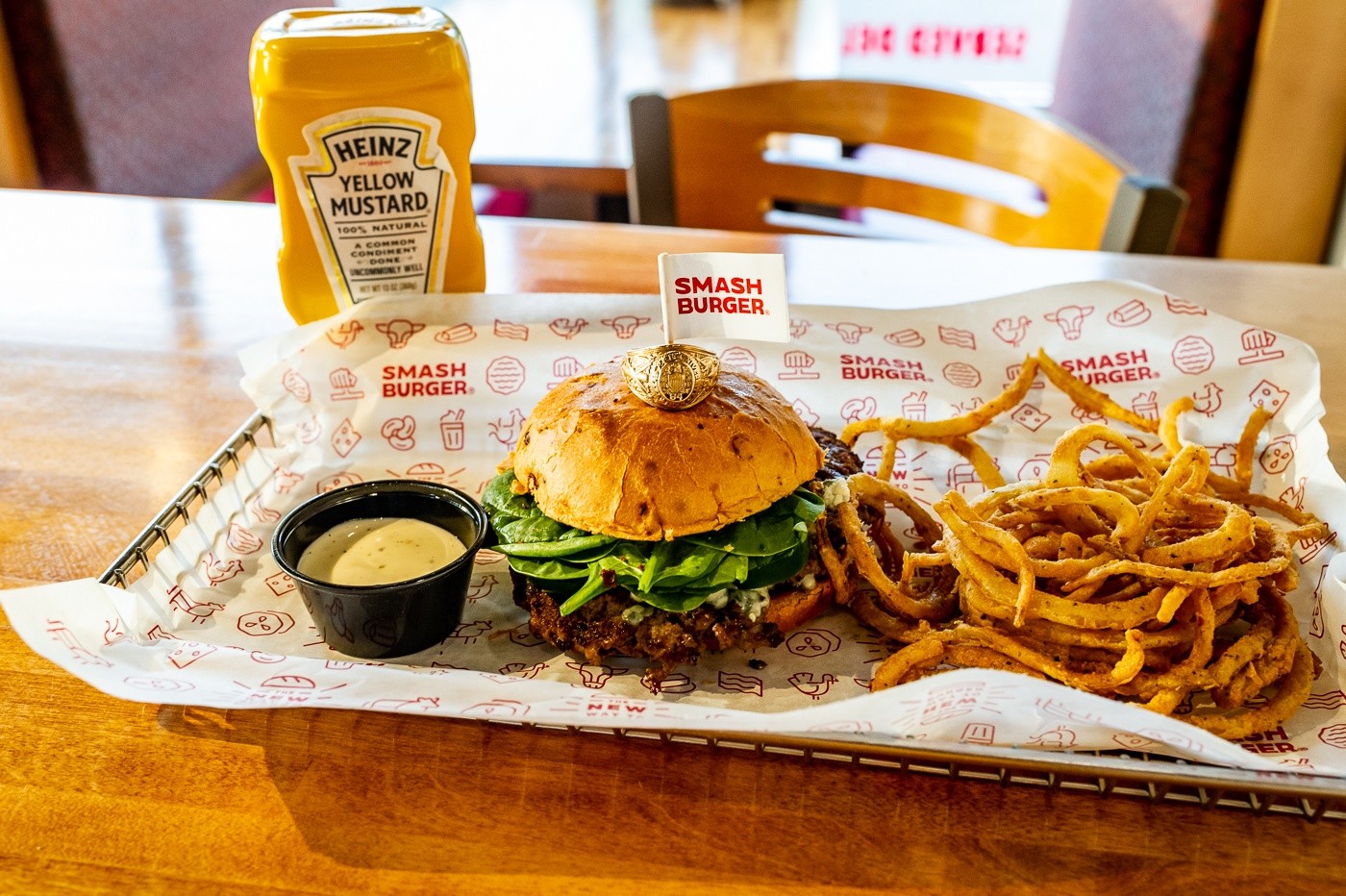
<box><xmin>0</xmin><ymin>186</ymin><xmax>1346</xmax><ymax>896</ymax></box>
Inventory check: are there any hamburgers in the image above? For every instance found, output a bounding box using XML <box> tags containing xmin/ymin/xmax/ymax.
<box><xmin>481</xmin><ymin>358</ymin><xmax>872</xmax><ymax>676</ymax></box>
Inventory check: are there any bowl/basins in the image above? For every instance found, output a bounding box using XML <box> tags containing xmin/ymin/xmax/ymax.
<box><xmin>271</xmin><ymin>479</ymin><xmax>489</xmax><ymax>659</ymax></box>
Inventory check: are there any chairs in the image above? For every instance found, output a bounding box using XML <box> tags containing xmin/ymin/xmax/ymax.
<box><xmin>626</xmin><ymin>78</ymin><xmax>1189</xmax><ymax>258</ymax></box>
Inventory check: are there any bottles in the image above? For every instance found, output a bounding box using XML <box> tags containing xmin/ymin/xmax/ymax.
<box><xmin>249</xmin><ymin>6</ymin><xmax>486</xmax><ymax>326</ymax></box>
